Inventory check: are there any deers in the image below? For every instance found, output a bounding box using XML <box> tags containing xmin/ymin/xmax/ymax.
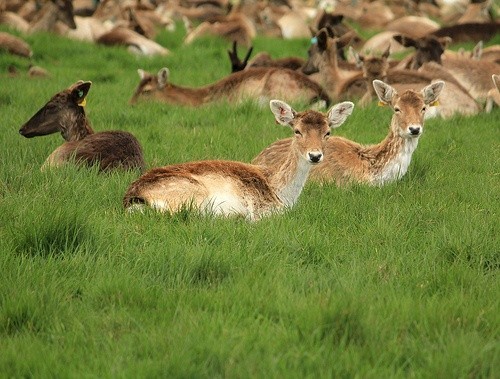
<box><xmin>251</xmin><ymin>80</ymin><xmax>445</xmax><ymax>186</ymax></box>
<box><xmin>122</xmin><ymin>100</ymin><xmax>354</xmax><ymax>222</ymax></box>
<box><xmin>18</xmin><ymin>80</ymin><xmax>143</xmax><ymax>174</ymax></box>
<box><xmin>0</xmin><ymin>0</ymin><xmax>500</xmax><ymax>120</ymax></box>
